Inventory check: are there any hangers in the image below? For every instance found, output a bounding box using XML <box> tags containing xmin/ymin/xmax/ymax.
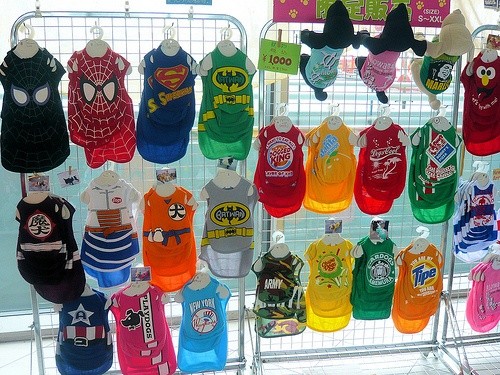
<box><xmin>0</xmin><ymin>22</ymin><xmax>500</xmax><ymax>312</ymax></box>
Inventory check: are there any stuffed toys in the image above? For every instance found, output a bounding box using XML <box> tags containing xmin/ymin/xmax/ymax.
<box><xmin>356</xmin><ymin>3</ymin><xmax>427</xmax><ymax>103</ymax></box>
<box><xmin>302</xmin><ymin>0</ymin><xmax>360</xmax><ymax>101</ymax></box>
<box><xmin>409</xmin><ymin>9</ymin><xmax>473</xmax><ymax>110</ymax></box>
